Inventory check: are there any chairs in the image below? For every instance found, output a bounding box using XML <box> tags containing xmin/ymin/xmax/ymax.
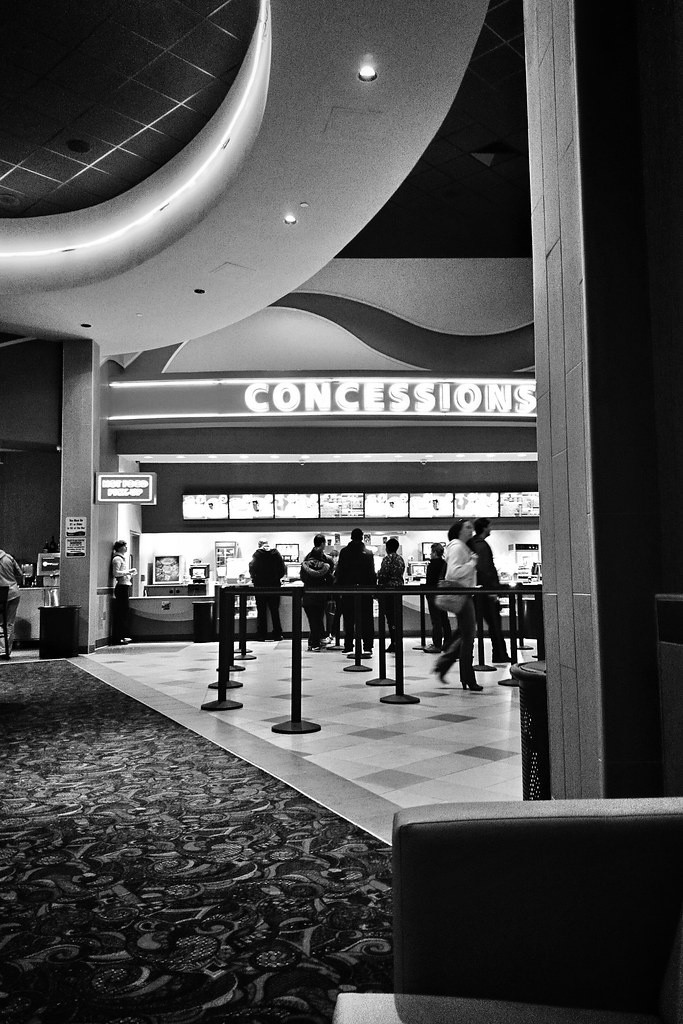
<box><xmin>389</xmin><ymin>796</ymin><xmax>683</xmax><ymax>1003</ymax></box>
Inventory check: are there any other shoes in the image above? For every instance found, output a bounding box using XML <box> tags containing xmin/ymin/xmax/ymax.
<box><xmin>0</xmin><ymin>655</ymin><xmax>7</xmax><ymax>660</ymax></box>
<box><xmin>113</xmin><ymin>638</ymin><xmax>132</xmax><ymax>645</ymax></box>
<box><xmin>342</xmin><ymin>644</ymin><xmax>353</xmax><ymax>653</ymax></box>
<box><xmin>492</xmin><ymin>657</ymin><xmax>512</xmax><ymax>663</ymax></box>
<box><xmin>385</xmin><ymin>647</ymin><xmax>395</xmax><ymax>652</ymax></box>
<box><xmin>274</xmin><ymin>636</ymin><xmax>283</xmax><ymax>640</ymax></box>
<box><xmin>365</xmin><ymin>649</ymin><xmax>372</xmax><ymax>652</ymax></box>
<box><xmin>424</xmin><ymin>644</ymin><xmax>441</xmax><ymax>653</ymax></box>
<box><xmin>307</xmin><ymin>645</ymin><xmax>321</xmax><ymax>652</ymax></box>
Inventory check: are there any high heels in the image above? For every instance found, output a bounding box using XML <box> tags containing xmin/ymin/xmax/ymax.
<box><xmin>463</xmin><ymin>680</ymin><xmax>483</xmax><ymax>691</ymax></box>
<box><xmin>435</xmin><ymin>666</ymin><xmax>448</xmax><ymax>683</ymax></box>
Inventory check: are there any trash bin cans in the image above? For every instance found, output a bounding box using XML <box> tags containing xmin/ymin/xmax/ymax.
<box><xmin>38</xmin><ymin>605</ymin><xmax>81</xmax><ymax>658</ymax></box>
<box><xmin>510</xmin><ymin>661</ymin><xmax>550</xmax><ymax>799</ymax></box>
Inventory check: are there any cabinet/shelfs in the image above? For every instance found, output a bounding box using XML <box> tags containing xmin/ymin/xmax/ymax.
<box><xmin>144</xmin><ymin>584</ymin><xmax>207</xmax><ymax>598</ymax></box>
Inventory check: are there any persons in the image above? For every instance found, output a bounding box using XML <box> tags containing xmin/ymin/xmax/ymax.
<box><xmin>112</xmin><ymin>540</ymin><xmax>137</xmax><ymax>645</ymax></box>
<box><xmin>0</xmin><ymin>549</ymin><xmax>25</xmax><ymax>658</ymax></box>
<box><xmin>300</xmin><ymin>529</ymin><xmax>375</xmax><ymax>654</ymax></box>
<box><xmin>430</xmin><ymin>519</ymin><xmax>484</xmax><ymax>691</ymax></box>
<box><xmin>378</xmin><ymin>538</ymin><xmax>405</xmax><ymax>652</ymax></box>
<box><xmin>466</xmin><ymin>516</ymin><xmax>515</xmax><ymax>663</ymax></box>
<box><xmin>249</xmin><ymin>538</ymin><xmax>288</xmax><ymax>641</ymax></box>
<box><xmin>423</xmin><ymin>542</ymin><xmax>450</xmax><ymax>654</ymax></box>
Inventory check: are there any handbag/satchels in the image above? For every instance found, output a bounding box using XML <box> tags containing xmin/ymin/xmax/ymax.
<box><xmin>435</xmin><ymin>580</ymin><xmax>467</xmax><ymax>613</ymax></box>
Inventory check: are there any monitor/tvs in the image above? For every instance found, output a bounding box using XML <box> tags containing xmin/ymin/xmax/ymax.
<box><xmin>190</xmin><ymin>566</ymin><xmax>207</xmax><ymax>579</ymax></box>
<box><xmin>410</xmin><ymin>564</ymin><xmax>427</xmax><ymax>576</ymax></box>
<box><xmin>286</xmin><ymin>565</ymin><xmax>302</xmax><ymax>580</ymax></box>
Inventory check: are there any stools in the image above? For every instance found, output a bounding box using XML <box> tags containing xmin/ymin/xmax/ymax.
<box><xmin>0</xmin><ymin>586</ymin><xmax>10</xmax><ymax>661</ymax></box>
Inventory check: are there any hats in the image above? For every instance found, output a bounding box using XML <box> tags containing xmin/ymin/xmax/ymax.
<box><xmin>114</xmin><ymin>540</ymin><xmax>126</xmax><ymax>549</ymax></box>
